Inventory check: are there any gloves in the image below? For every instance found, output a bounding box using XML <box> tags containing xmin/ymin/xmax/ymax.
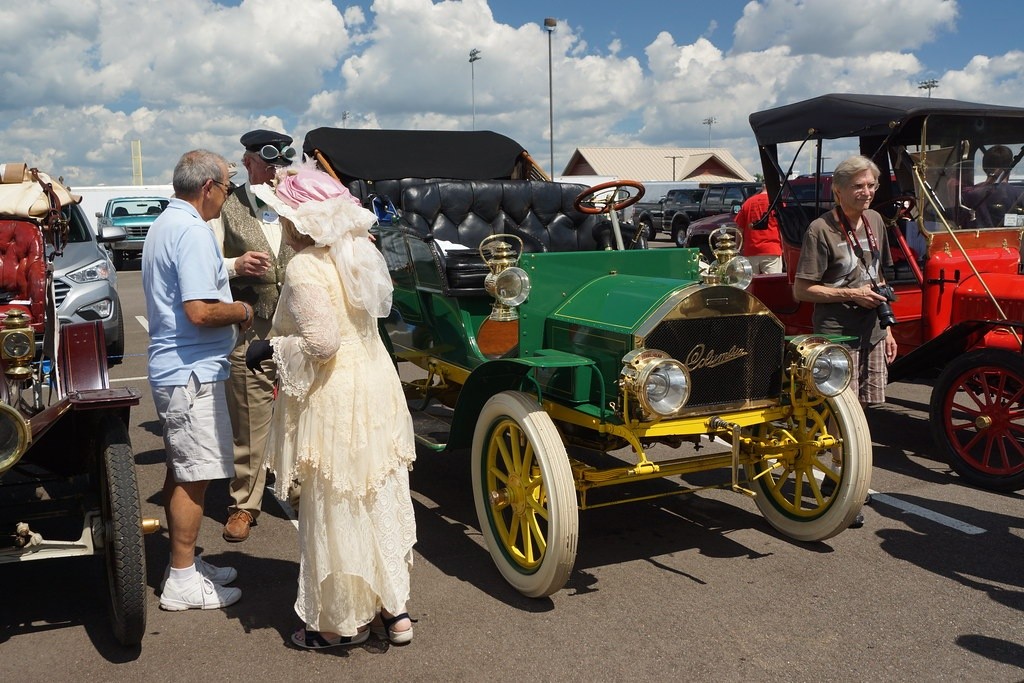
<box><xmin>244</xmin><ymin>338</ymin><xmax>273</xmax><ymax>376</ymax></box>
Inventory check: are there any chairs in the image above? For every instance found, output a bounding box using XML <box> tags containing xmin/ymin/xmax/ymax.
<box><xmin>0</xmin><ymin>219</ymin><xmax>47</xmax><ymax>334</ymax></box>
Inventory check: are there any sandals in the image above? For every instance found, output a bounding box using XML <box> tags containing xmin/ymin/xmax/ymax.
<box><xmin>369</xmin><ymin>610</ymin><xmax>418</xmax><ymax>644</ymax></box>
<box><xmin>291</xmin><ymin>622</ymin><xmax>371</xmax><ymax>649</ymax></box>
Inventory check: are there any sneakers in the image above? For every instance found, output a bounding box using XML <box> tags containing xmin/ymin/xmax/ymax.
<box><xmin>159</xmin><ymin>571</ymin><xmax>241</xmax><ymax>611</ymax></box>
<box><xmin>222</xmin><ymin>510</ymin><xmax>254</xmax><ymax>541</ymax></box>
<box><xmin>161</xmin><ymin>553</ymin><xmax>237</xmax><ymax>592</ymax></box>
<box><xmin>288</xmin><ymin>479</ymin><xmax>302</xmax><ymax>510</ymax></box>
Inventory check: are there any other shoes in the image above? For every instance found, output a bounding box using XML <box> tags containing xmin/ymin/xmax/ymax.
<box><xmin>850</xmin><ymin>512</ymin><xmax>864</xmax><ymax>528</ymax></box>
<box><xmin>820</xmin><ymin>475</ymin><xmax>871</xmax><ymax>505</ymax></box>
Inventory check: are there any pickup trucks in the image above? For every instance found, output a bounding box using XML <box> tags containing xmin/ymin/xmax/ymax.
<box><xmin>662</xmin><ymin>182</ymin><xmax>764</xmax><ymax>249</ymax></box>
<box><xmin>632</xmin><ymin>188</ymin><xmax>706</xmax><ymax>241</ymax></box>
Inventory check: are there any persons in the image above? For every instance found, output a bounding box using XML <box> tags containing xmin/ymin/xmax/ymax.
<box><xmin>957</xmin><ymin>145</ymin><xmax>1024</xmax><ymax>230</ymax></box>
<box><xmin>206</xmin><ymin>129</ymin><xmax>375</xmax><ymax>542</ymax></box>
<box><xmin>245</xmin><ymin>153</ymin><xmax>419</xmax><ymax>649</ymax></box>
<box><xmin>792</xmin><ymin>154</ymin><xmax>899</xmax><ymax>524</ymax></box>
<box><xmin>735</xmin><ymin>178</ymin><xmax>784</xmax><ymax>276</ymax></box>
<box><xmin>143</xmin><ymin>149</ymin><xmax>255</xmax><ymax>611</ymax></box>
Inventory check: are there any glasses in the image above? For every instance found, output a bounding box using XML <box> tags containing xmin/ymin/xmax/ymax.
<box><xmin>201</xmin><ymin>178</ymin><xmax>235</xmax><ymax>197</ymax></box>
<box><xmin>254</xmin><ymin>144</ymin><xmax>297</xmax><ymax>161</ymax></box>
<box><xmin>836</xmin><ymin>180</ymin><xmax>880</xmax><ymax>192</ymax></box>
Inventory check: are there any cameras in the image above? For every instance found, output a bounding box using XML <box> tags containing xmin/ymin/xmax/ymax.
<box><xmin>871</xmin><ymin>284</ymin><xmax>898</xmax><ymax>329</ymax></box>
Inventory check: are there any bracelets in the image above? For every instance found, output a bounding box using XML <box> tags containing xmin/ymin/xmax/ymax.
<box><xmin>235</xmin><ymin>300</ymin><xmax>249</xmax><ymax>305</ymax></box>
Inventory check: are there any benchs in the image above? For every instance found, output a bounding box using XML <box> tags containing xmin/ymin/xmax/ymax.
<box><xmin>782</xmin><ymin>204</ymin><xmax>831</xmax><ymax>248</ymax></box>
<box><xmin>401</xmin><ymin>179</ymin><xmax>638</xmax><ymax>287</ymax></box>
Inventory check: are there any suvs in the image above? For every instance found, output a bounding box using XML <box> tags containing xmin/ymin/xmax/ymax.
<box><xmin>0</xmin><ymin>157</ymin><xmax>162</xmax><ymax>649</ymax></box>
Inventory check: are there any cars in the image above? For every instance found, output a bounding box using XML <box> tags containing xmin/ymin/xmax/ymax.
<box><xmin>744</xmin><ymin>93</ymin><xmax>1024</xmax><ymax>495</ymax></box>
<box><xmin>300</xmin><ymin>124</ymin><xmax>876</xmax><ymax>602</ymax></box>
<box><xmin>685</xmin><ymin>174</ymin><xmax>898</xmax><ymax>265</ymax></box>
<box><xmin>94</xmin><ymin>195</ymin><xmax>172</xmax><ymax>271</ymax></box>
<box><xmin>0</xmin><ymin>193</ymin><xmax>128</xmax><ymax>365</ymax></box>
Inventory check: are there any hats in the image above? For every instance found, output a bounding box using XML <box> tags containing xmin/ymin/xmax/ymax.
<box><xmin>249</xmin><ymin>153</ymin><xmax>394</xmax><ymax>318</ymax></box>
<box><xmin>240</xmin><ymin>130</ymin><xmax>292</xmax><ymax>152</ymax></box>
